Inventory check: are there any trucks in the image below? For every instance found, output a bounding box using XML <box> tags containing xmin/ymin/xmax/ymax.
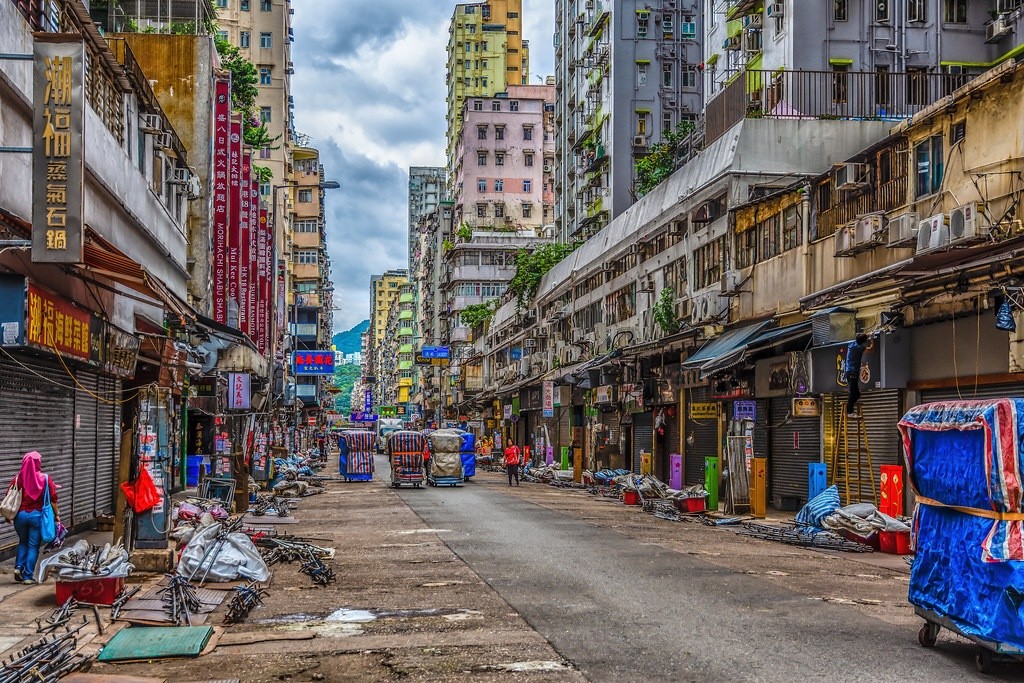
<box><xmin>376</xmin><ymin>418</ymin><xmax>404</xmax><ymax>454</ymax></box>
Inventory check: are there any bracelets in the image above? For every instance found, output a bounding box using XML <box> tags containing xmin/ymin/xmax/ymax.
<box><xmin>56</xmin><ymin>511</ymin><xmax>60</xmax><ymax>516</ymax></box>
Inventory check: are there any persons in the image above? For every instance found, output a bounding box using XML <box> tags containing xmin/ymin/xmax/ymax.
<box><xmin>476</xmin><ymin>436</ymin><xmax>493</xmax><ymax>455</ymax></box>
<box><xmin>5</xmin><ymin>451</ymin><xmax>60</xmax><ymax>585</ymax></box>
<box><xmin>845</xmin><ymin>332</ymin><xmax>875</xmax><ymax>418</ymax></box>
<box><xmin>319</xmin><ymin>436</ymin><xmax>328</xmax><ymax>462</ymax></box>
<box><xmin>503</xmin><ymin>438</ymin><xmax>520</xmax><ymax>486</ymax></box>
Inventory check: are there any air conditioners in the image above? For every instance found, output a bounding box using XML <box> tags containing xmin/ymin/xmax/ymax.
<box><xmin>137</xmin><ymin>112</ymin><xmax>193</xmax><ymax>196</ymax></box>
<box><xmin>505</xmin><ymin>216</ymin><xmax>513</xmax><ymax>221</ymax></box>
<box><xmin>986</xmin><ymin>17</ymin><xmax>1007</xmax><ymax>43</ymax></box>
<box><xmin>543</xmin><ymin>165</ymin><xmax>552</xmax><ymax>173</ymax></box>
<box><xmin>724</xmin><ymin>3</ymin><xmax>784</xmax><ymax>50</ymax></box>
<box><xmin>638</xmin><ymin>12</ymin><xmax>648</xmax><ymax>21</ymax></box>
<box><xmin>633</xmin><ymin>135</ymin><xmax>646</xmax><ymax>147</ymax></box>
<box><xmin>691</xmin><ymin>269</ymin><xmax>741</xmax><ymax>325</ymax></box>
<box><xmin>834</xmin><ymin>162</ymin><xmax>870</xmax><ymax>191</ymax></box>
<box><xmin>495</xmin><ymin>300</ymin><xmax>582</xmax><ymax>377</ymax></box>
<box><xmin>833</xmin><ymin>200</ymin><xmax>991</xmax><ymax>256</ymax></box>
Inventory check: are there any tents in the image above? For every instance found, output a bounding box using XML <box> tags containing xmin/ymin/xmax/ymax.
<box><xmin>763</xmin><ymin>99</ymin><xmax>817</xmax><ymax>120</ymax></box>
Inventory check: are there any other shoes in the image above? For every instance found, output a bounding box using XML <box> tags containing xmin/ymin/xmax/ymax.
<box><xmin>847</xmin><ymin>413</ymin><xmax>861</xmax><ymax>418</ymax></box>
<box><xmin>14</xmin><ymin>566</ymin><xmax>23</xmax><ymax>581</ymax></box>
<box><xmin>24</xmin><ymin>578</ymin><xmax>36</xmax><ymax>584</ymax></box>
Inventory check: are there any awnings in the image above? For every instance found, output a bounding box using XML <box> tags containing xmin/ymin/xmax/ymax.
<box><xmin>681</xmin><ymin>319</ymin><xmax>774</xmax><ymax>368</ymax></box>
<box><xmin>168</xmin><ymin>310</ymin><xmax>258</xmax><ymax>353</ymax></box>
<box><xmin>800</xmin><ymin>231</ymin><xmax>1024</xmax><ymax>309</ymax></box>
<box><xmin>494</xmin><ymin>372</ymin><xmax>549</xmax><ymax>397</ymax></box>
<box><xmin>622</xmin><ymin>327</ymin><xmax>705</xmax><ymax>378</ymax></box>
<box><xmin>0</xmin><ymin>207</ymin><xmax>198</xmax><ymax>318</ymax></box>
<box><xmin>700</xmin><ymin>318</ymin><xmax>811</xmax><ymax>378</ymax></box>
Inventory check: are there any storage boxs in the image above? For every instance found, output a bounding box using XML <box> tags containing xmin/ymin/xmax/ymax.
<box><xmin>623</xmin><ymin>491</ymin><xmax>636</xmax><ymax>505</ymax></box>
<box><xmin>187</xmin><ymin>454</ymin><xmax>211</xmax><ymax>487</ymax></box>
<box><xmin>845</xmin><ymin>528</ymin><xmax>916</xmax><ymax>556</ymax></box>
<box><xmin>56</xmin><ymin>577</ymin><xmax>125</xmax><ymax>607</ymax></box>
<box><xmin>680</xmin><ymin>496</ymin><xmax>706</xmax><ymax>512</ymax></box>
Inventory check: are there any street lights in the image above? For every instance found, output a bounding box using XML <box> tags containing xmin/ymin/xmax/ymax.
<box><xmin>263</xmin><ymin>180</ymin><xmax>345</xmax><ymax>486</ymax></box>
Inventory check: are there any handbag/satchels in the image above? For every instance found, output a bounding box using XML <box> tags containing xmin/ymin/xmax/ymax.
<box><xmin>119</xmin><ymin>461</ymin><xmax>162</xmax><ymax>515</ymax></box>
<box><xmin>0</xmin><ymin>474</ymin><xmax>24</xmax><ymax>519</ymax></box>
<box><xmin>39</xmin><ymin>474</ymin><xmax>56</xmax><ymax>542</ymax></box>
